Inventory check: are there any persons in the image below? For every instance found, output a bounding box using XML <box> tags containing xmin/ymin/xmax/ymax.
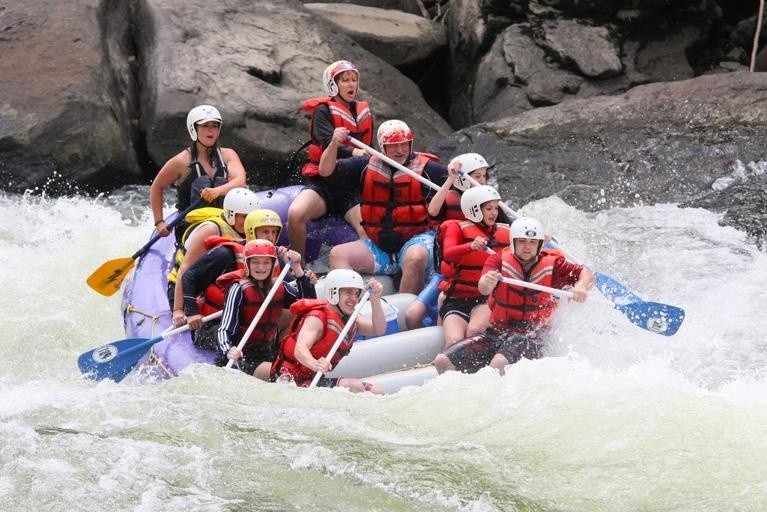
<box><xmin>319</xmin><ymin>120</ymin><xmax>444</xmax><ymax>294</ymax></box>
<box><xmin>182</xmin><ymin>210</ymin><xmax>317</xmax><ymax>351</ymax></box>
<box><xmin>217</xmin><ymin>240</ymin><xmax>318</xmax><ymax>379</ymax></box>
<box><xmin>150</xmin><ymin>106</ymin><xmax>248</xmax><ymax>235</ymax></box>
<box><xmin>287</xmin><ymin>60</ymin><xmax>373</xmax><ymax>266</ymax></box>
<box><xmin>268</xmin><ymin>268</ymin><xmax>387</xmax><ymax>392</ymax></box>
<box><xmin>440</xmin><ymin>185</ymin><xmax>519</xmax><ymax>346</ymax></box>
<box><xmin>166</xmin><ymin>188</ymin><xmax>258</xmax><ymax>326</ymax></box>
<box><xmin>404</xmin><ymin>151</ymin><xmax>491</xmax><ymax>328</ymax></box>
<box><xmin>436</xmin><ymin>216</ymin><xmax>595</xmax><ymax>375</ymax></box>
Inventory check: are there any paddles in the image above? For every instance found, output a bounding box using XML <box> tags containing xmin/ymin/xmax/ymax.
<box><xmin>77</xmin><ymin>309</ymin><xmax>225</xmax><ymax>379</ymax></box>
<box><xmin>86</xmin><ymin>189</ymin><xmax>206</xmax><ymax>297</ymax></box>
<box><xmin>486</xmin><ymin>246</ymin><xmax>645</xmax><ymax>305</ymax></box>
<box><xmin>500</xmin><ymin>276</ymin><xmax>684</xmax><ymax>336</ymax></box>
<box><xmin>458</xmin><ymin>169</ymin><xmax>560</xmax><ymax>251</ymax></box>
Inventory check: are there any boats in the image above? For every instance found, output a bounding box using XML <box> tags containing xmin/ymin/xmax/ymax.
<box><xmin>120</xmin><ymin>186</ymin><xmax>580</xmax><ymax>387</ymax></box>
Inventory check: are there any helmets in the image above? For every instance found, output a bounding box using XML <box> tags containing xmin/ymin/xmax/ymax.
<box><xmin>376</xmin><ymin>119</ymin><xmax>546</xmax><ymax>260</ymax></box>
<box><xmin>186</xmin><ymin>105</ymin><xmax>224</xmax><ymax>142</ymax></box>
<box><xmin>223</xmin><ymin>188</ymin><xmax>365</xmax><ymax>305</ymax></box>
<box><xmin>322</xmin><ymin>60</ymin><xmax>360</xmax><ymax>98</ymax></box>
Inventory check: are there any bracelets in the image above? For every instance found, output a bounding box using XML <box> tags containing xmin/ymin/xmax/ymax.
<box><xmin>154</xmin><ymin>220</ymin><xmax>165</xmax><ymax>226</ymax></box>
